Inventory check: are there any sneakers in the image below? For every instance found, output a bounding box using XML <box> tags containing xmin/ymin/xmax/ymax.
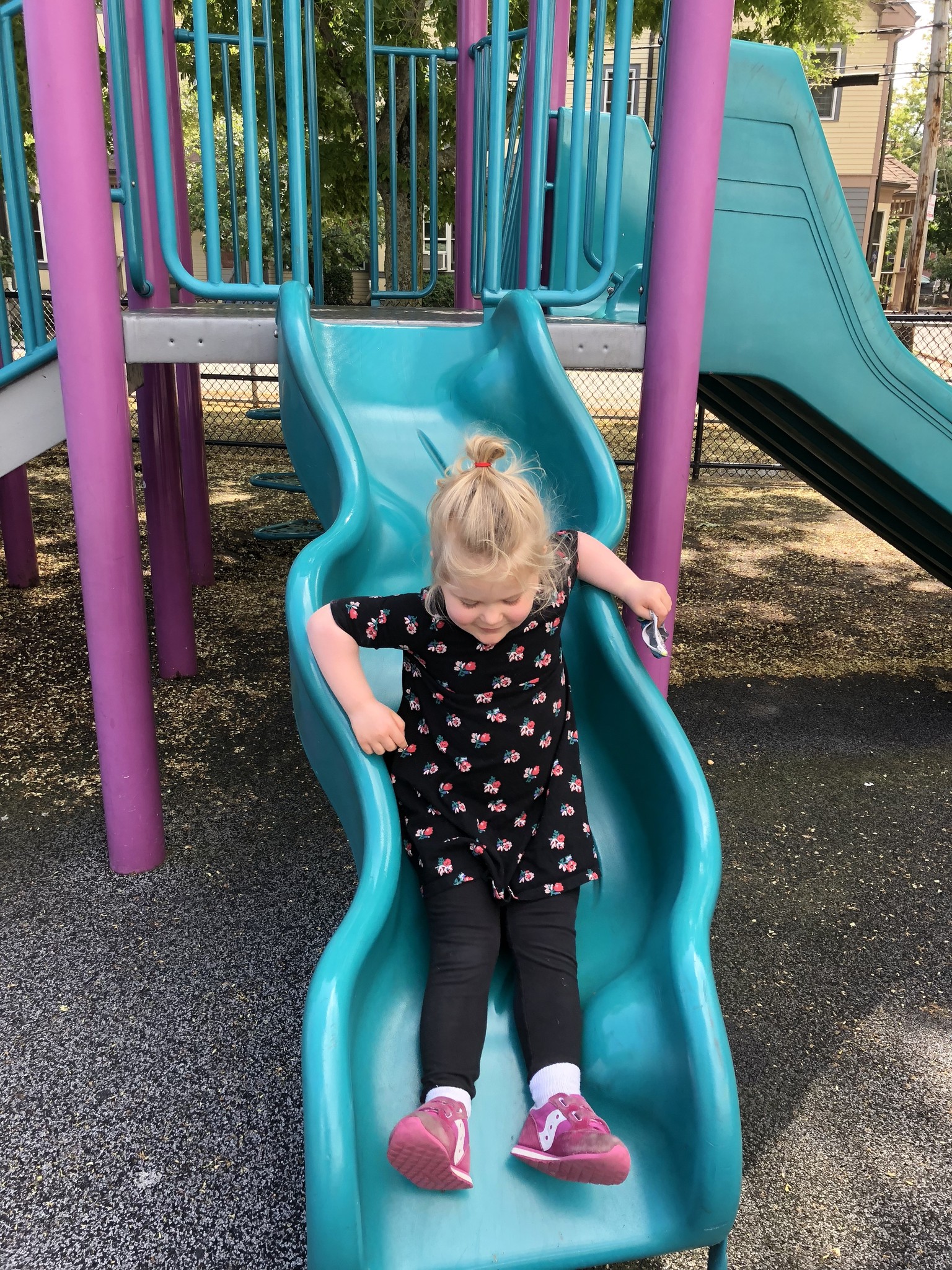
<box><xmin>509</xmin><ymin>1091</ymin><xmax>630</xmax><ymax>1185</ymax></box>
<box><xmin>386</xmin><ymin>1096</ymin><xmax>473</xmax><ymax>1190</ymax></box>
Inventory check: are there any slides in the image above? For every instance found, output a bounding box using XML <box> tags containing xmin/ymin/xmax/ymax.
<box><xmin>569</xmin><ymin>0</ymin><xmax>951</xmax><ymax>585</ymax></box>
<box><xmin>279</xmin><ymin>288</ymin><xmax>744</xmax><ymax>1270</ymax></box>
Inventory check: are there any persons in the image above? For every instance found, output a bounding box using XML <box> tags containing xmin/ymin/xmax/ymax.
<box><xmin>306</xmin><ymin>432</ymin><xmax>671</xmax><ymax>1191</ymax></box>
